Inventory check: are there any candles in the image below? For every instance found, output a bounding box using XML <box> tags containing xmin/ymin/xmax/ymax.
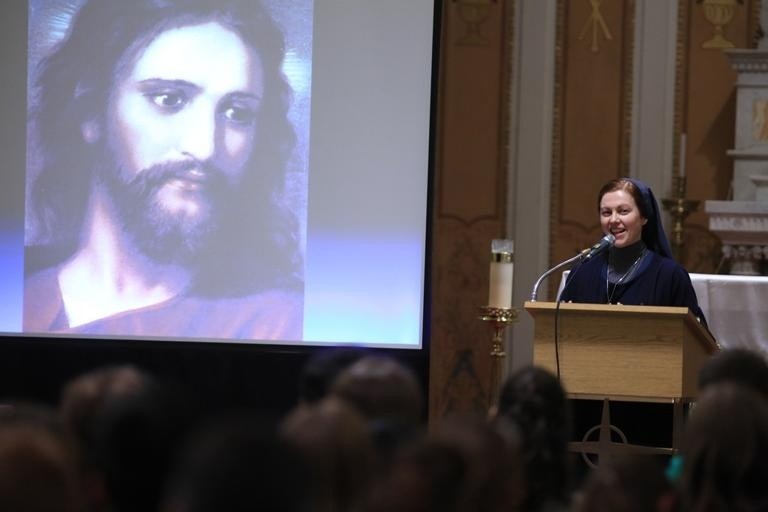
<box><xmin>678</xmin><ymin>134</ymin><xmax>687</xmax><ymax>177</ymax></box>
<box><xmin>487</xmin><ymin>239</ymin><xmax>516</xmax><ymax>311</ymax></box>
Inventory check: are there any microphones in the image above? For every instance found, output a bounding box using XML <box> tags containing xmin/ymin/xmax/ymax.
<box><xmin>582</xmin><ymin>234</ymin><xmax>615</xmax><ymax>264</ymax></box>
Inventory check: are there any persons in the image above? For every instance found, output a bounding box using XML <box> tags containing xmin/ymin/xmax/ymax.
<box><xmin>24</xmin><ymin>2</ymin><xmax>314</xmax><ymax>339</ymax></box>
<box><xmin>557</xmin><ymin>177</ymin><xmax>713</xmax><ymax>470</ymax></box>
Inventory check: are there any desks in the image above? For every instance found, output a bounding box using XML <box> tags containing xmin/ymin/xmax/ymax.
<box><xmin>559</xmin><ymin>270</ymin><xmax>767</xmax><ymax>356</ymax></box>
